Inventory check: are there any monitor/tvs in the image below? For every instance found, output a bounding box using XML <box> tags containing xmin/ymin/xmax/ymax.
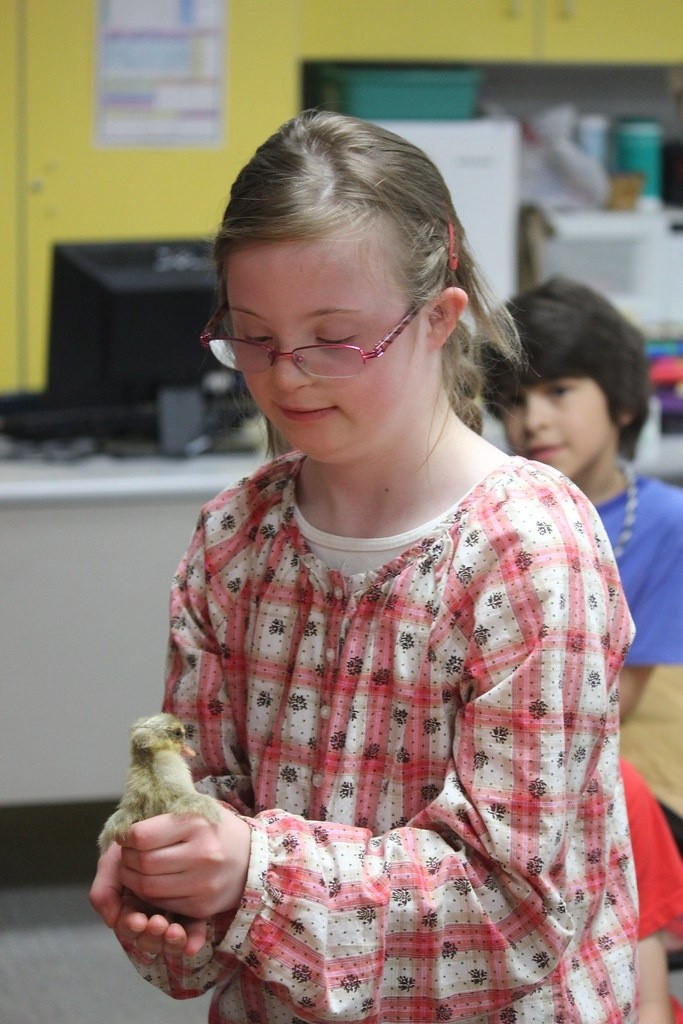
<box><xmin>48</xmin><ymin>238</ymin><xmax>261</xmax><ymax>457</ymax></box>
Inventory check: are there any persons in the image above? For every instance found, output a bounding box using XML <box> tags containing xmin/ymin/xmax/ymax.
<box><xmin>472</xmin><ymin>276</ymin><xmax>683</xmax><ymax>857</ymax></box>
<box><xmin>617</xmin><ymin>754</ymin><xmax>682</xmax><ymax>1024</ymax></box>
<box><xmin>88</xmin><ymin>106</ymin><xmax>641</xmax><ymax>1022</ymax></box>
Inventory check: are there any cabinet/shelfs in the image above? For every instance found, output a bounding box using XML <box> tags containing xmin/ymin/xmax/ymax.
<box><xmin>0</xmin><ymin>0</ymin><xmax>683</xmax><ymax>391</ymax></box>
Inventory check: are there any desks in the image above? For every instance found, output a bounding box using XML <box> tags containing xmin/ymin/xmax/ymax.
<box><xmin>1</xmin><ymin>417</ymin><xmax>274</xmax><ymax>886</ymax></box>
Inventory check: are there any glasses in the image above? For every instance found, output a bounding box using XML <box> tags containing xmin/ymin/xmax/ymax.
<box><xmin>200</xmin><ymin>293</ymin><xmax>438</xmax><ymax>377</ymax></box>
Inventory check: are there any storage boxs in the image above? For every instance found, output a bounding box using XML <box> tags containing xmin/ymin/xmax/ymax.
<box><xmin>315</xmin><ymin>62</ymin><xmax>480</xmax><ymax>119</ymax></box>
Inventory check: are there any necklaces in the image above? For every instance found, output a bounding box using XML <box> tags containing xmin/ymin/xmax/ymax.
<box><xmin>612</xmin><ymin>457</ymin><xmax>636</xmax><ymax>559</ymax></box>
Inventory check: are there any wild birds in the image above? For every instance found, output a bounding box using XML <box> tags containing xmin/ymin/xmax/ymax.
<box><xmin>96</xmin><ymin>711</ymin><xmax>221</xmax><ymax>853</ymax></box>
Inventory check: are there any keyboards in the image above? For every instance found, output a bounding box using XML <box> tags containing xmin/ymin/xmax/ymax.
<box><xmin>0</xmin><ymin>395</ymin><xmax>255</xmax><ymax>441</ymax></box>
